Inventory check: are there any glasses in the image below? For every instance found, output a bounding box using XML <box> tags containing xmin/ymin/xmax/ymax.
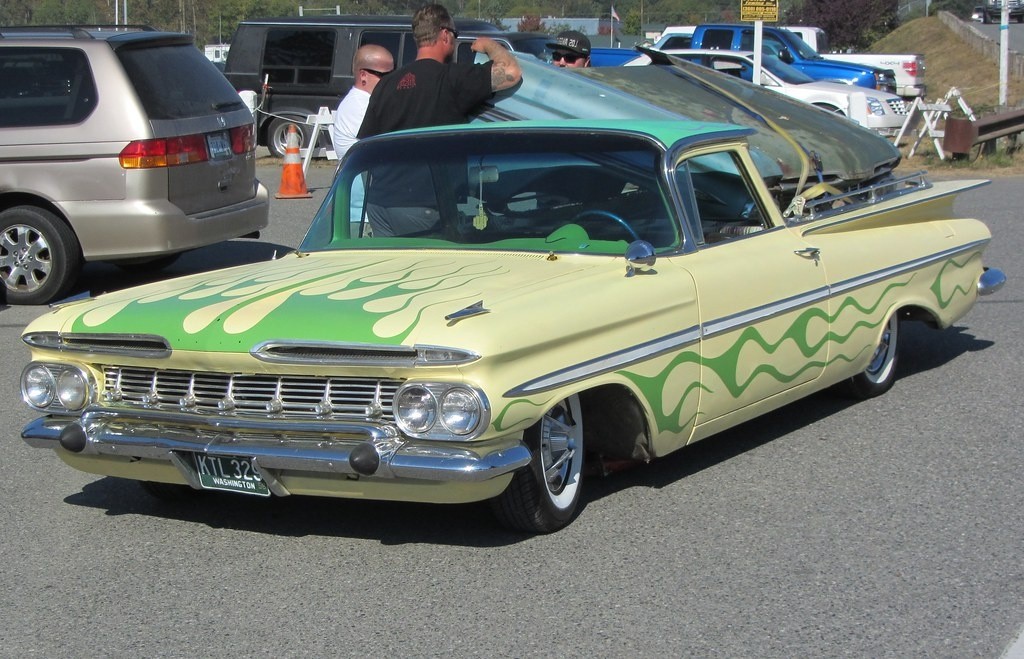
<box><xmin>551</xmin><ymin>51</ymin><xmax>588</xmax><ymax>62</ymax></box>
<box><xmin>361</xmin><ymin>68</ymin><xmax>392</xmax><ymax>80</ymax></box>
<box><xmin>440</xmin><ymin>25</ymin><xmax>460</xmax><ymax>39</ymax></box>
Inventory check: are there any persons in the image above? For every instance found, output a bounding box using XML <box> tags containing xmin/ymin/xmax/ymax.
<box><xmin>355</xmin><ymin>3</ymin><xmax>523</xmax><ymax>241</ymax></box>
<box><xmin>331</xmin><ymin>44</ymin><xmax>396</xmax><ymax>241</ymax></box>
<box><xmin>551</xmin><ymin>31</ymin><xmax>592</xmax><ymax>70</ymax></box>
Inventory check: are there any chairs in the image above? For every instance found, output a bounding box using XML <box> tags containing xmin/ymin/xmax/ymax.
<box><xmin>644</xmin><ymin>171</ymin><xmax>757</xmax><ymax>248</ymax></box>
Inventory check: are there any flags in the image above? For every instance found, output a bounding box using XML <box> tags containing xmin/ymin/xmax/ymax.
<box><xmin>611</xmin><ymin>3</ymin><xmax>619</xmax><ymax>24</ymax></box>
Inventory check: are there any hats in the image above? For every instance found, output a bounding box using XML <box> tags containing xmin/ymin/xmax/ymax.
<box><xmin>546</xmin><ymin>30</ymin><xmax>591</xmax><ymax>55</ymax></box>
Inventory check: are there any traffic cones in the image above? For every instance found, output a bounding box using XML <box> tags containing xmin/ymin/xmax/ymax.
<box><xmin>274</xmin><ymin>124</ymin><xmax>313</xmax><ymax>198</ymax></box>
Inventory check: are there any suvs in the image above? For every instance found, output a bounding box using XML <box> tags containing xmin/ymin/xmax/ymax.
<box><xmin>0</xmin><ymin>24</ymin><xmax>270</xmax><ymax>305</ymax></box>
<box><xmin>622</xmin><ymin>49</ymin><xmax>910</xmax><ymax>139</ymax></box>
<box><xmin>451</xmin><ymin>28</ymin><xmax>559</xmax><ymax>66</ymax></box>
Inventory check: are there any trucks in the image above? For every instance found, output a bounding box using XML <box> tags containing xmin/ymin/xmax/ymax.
<box><xmin>585</xmin><ymin>24</ymin><xmax>925</xmax><ymax>95</ymax></box>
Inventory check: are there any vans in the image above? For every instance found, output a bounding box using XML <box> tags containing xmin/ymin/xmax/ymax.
<box><xmin>224</xmin><ymin>14</ymin><xmax>502</xmax><ymax>158</ymax></box>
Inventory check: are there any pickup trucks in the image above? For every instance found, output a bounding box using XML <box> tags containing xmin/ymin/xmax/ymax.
<box><xmin>18</xmin><ymin>118</ymin><xmax>1005</xmax><ymax>535</ymax></box>
